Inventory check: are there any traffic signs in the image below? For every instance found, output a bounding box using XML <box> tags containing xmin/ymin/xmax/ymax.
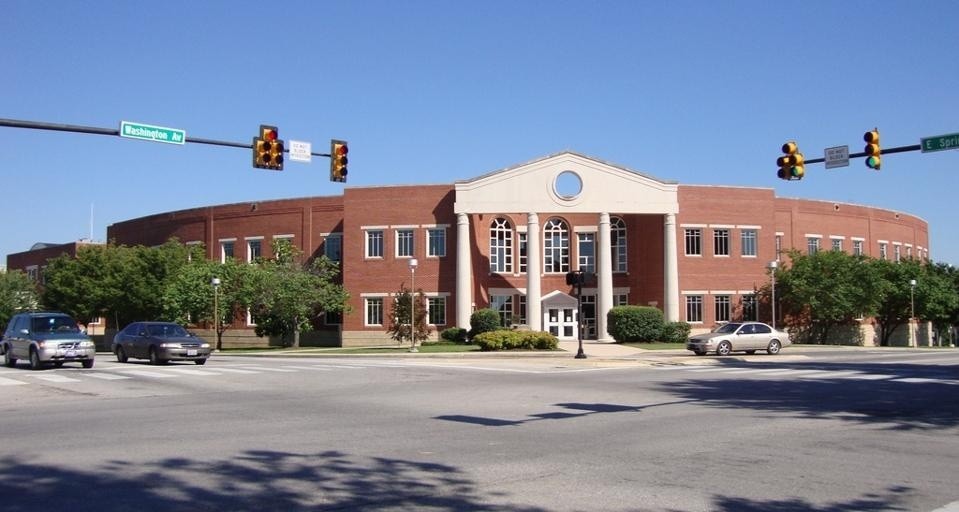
<box><xmin>122</xmin><ymin>123</ymin><xmax>184</xmax><ymax>144</ymax></box>
<box><xmin>921</xmin><ymin>133</ymin><xmax>959</xmax><ymax>152</ymax></box>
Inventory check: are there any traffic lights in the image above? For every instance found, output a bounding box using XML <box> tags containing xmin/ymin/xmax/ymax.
<box><xmin>332</xmin><ymin>139</ymin><xmax>348</xmax><ymax>183</ymax></box>
<box><xmin>565</xmin><ymin>271</ymin><xmax>576</xmax><ymax>285</ymax></box>
<box><xmin>254</xmin><ymin>137</ymin><xmax>271</xmax><ymax>170</ymax></box>
<box><xmin>776</xmin><ymin>156</ymin><xmax>789</xmax><ymax>180</ymax></box>
<box><xmin>781</xmin><ymin>141</ymin><xmax>797</xmax><ymax>155</ymax></box>
<box><xmin>863</xmin><ymin>130</ymin><xmax>881</xmax><ymax>171</ymax></box>
<box><xmin>260</xmin><ymin>123</ymin><xmax>279</xmax><ymax>142</ymax></box>
<box><xmin>580</xmin><ymin>272</ymin><xmax>593</xmax><ymax>284</ymax></box>
<box><xmin>789</xmin><ymin>153</ymin><xmax>804</xmax><ymax>178</ymax></box>
<box><xmin>271</xmin><ymin>139</ymin><xmax>283</xmax><ymax>171</ymax></box>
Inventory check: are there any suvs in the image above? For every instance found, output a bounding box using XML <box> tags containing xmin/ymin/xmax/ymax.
<box><xmin>0</xmin><ymin>312</ymin><xmax>96</xmax><ymax>370</ymax></box>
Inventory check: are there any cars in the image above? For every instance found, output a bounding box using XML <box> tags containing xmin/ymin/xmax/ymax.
<box><xmin>687</xmin><ymin>321</ymin><xmax>791</xmax><ymax>356</ymax></box>
<box><xmin>111</xmin><ymin>321</ymin><xmax>211</xmax><ymax>365</ymax></box>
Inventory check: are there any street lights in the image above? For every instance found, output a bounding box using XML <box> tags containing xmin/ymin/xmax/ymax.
<box><xmin>212</xmin><ymin>277</ymin><xmax>220</xmax><ymax>352</ymax></box>
<box><xmin>908</xmin><ymin>280</ymin><xmax>916</xmax><ymax>348</ymax></box>
<box><xmin>409</xmin><ymin>259</ymin><xmax>418</xmax><ymax>352</ymax></box>
<box><xmin>769</xmin><ymin>261</ymin><xmax>777</xmax><ymax>328</ymax></box>
